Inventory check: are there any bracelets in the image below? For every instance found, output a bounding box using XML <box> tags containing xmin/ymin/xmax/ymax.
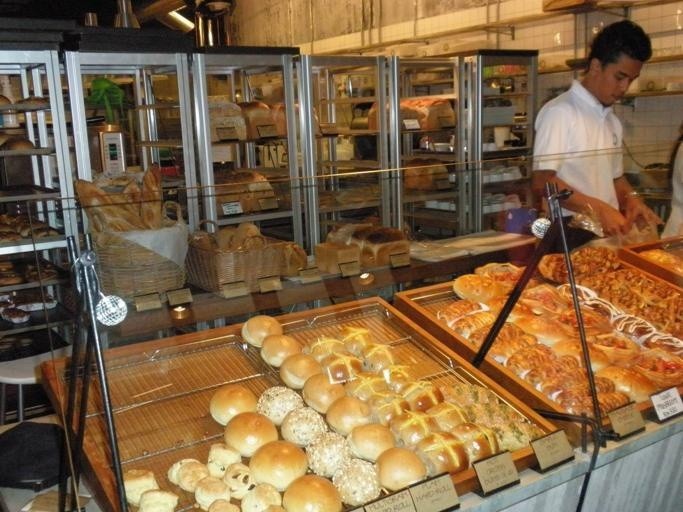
<box><xmin>623</xmin><ymin>191</ymin><xmax>640</xmax><ymax>199</ymax></box>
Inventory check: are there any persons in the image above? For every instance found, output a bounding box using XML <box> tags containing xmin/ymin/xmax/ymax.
<box><xmin>660</xmin><ymin>141</ymin><xmax>683</xmax><ymax>239</ymax></box>
<box><xmin>531</xmin><ymin>19</ymin><xmax>665</xmax><ymax>253</ymax></box>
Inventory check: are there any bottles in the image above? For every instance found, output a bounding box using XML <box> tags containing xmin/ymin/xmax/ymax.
<box><xmin>0</xmin><ymin>110</ymin><xmax>20</xmax><ymax>130</ymax></box>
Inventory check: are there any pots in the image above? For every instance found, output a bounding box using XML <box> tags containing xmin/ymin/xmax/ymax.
<box><xmin>636</xmin><ymin>166</ymin><xmax>672</xmax><ymax>190</ymax></box>
<box><xmin>483</xmin><ymin>77</ymin><xmax>515</xmax><ymax>95</ymax></box>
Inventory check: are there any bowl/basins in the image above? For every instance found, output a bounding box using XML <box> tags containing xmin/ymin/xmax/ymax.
<box><xmin>480</xmin><ymin>105</ymin><xmax>514</xmax><ymax>128</ymax></box>
<box><xmin>159</xmin><ymin>165</ymin><xmax>179</xmax><ymax>178</ymax></box>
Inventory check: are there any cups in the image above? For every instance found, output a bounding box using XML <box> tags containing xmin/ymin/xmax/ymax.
<box><xmin>666</xmin><ymin>83</ymin><xmax>681</xmax><ymax>92</ymax></box>
<box><xmin>424</xmin><ymin>137</ymin><xmax>521</xmax><ymax>216</ymax></box>
<box><xmin>514</xmin><ymin>112</ymin><xmax>525</xmax><ymax>116</ymax></box>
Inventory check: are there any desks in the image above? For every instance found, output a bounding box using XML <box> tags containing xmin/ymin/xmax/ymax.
<box><xmin>101</xmin><ymin>227</ymin><xmax>534</xmax><ymax>335</ymax></box>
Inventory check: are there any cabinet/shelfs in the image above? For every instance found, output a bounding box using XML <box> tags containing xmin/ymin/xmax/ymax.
<box><xmin>181</xmin><ymin>50</ymin><xmax>312</xmax><ymax>262</ymax></box>
<box><xmin>1</xmin><ymin>48</ymin><xmax>198</xmax><ymax>336</ymax></box>
<box><xmin>295</xmin><ymin>52</ymin><xmax>534</xmax><ymax>247</ymax></box>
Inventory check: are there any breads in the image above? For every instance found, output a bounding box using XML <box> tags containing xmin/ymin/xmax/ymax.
<box><xmin>2</xmin><ymin>212</ymin><xmax>58</xmax><ymax>241</ymax></box>
<box><xmin>640</xmin><ymin>249</ymin><xmax>680</xmax><ymax>272</ymax></box>
<box><xmin>124</xmin><ymin>383</ymin><xmax>382</xmax><ymax>510</ymax></box>
<box><xmin>191</xmin><ymin>222</ymin><xmax>264</xmax><ymax>250</ymax></box>
<box><xmin>436</xmin><ymin>263</ymin><xmax>681</xmax><ymax>421</ymax></box>
<box><xmin>2</xmin><ymin>260</ymin><xmax>53</xmax><ymax>285</ymax></box>
<box><xmin>368</xmin><ymin>98</ymin><xmax>456</xmax><ymax>131</ymax></box>
<box><xmin>2</xmin><ymin>290</ymin><xmax>57</xmax><ymax>323</ymax></box>
<box><xmin>210</xmin><ymin>102</ymin><xmax>322</xmax><ymax>141</ymax></box>
<box><xmin>16</xmin><ymin>96</ymin><xmax>49</xmax><ymax>104</ymax></box>
<box><xmin>241</xmin><ymin>316</ymin><xmax>543</xmax><ymax>492</ymax></box>
<box><xmin>404</xmin><ymin>158</ymin><xmax>451</xmax><ymax>190</ymax></box>
<box><xmin>281</xmin><ymin>184</ymin><xmax>382</xmax><ymax>210</ymax></box>
<box><xmin>2</xmin><ymin>95</ymin><xmax>11</xmax><ymax>104</ymax></box>
<box><xmin>265</xmin><ymin>237</ymin><xmax>308</xmax><ymax>276</ymax></box>
<box><xmin>214</xmin><ymin>171</ymin><xmax>277</xmax><ymax>215</ymax></box>
<box><xmin>536</xmin><ymin>248</ymin><xmax>681</xmax><ymax>337</ymax></box>
<box><xmin>2</xmin><ymin>336</ymin><xmax>32</xmax><ymax>352</ymax></box>
<box><xmin>314</xmin><ymin>223</ymin><xmax>411</xmax><ymax>275</ymax></box>
<box><xmin>6</xmin><ymin>137</ymin><xmax>34</xmax><ymax>150</ymax></box>
<box><xmin>75</xmin><ymin>165</ymin><xmax>163</xmax><ymax>231</ymax></box>
